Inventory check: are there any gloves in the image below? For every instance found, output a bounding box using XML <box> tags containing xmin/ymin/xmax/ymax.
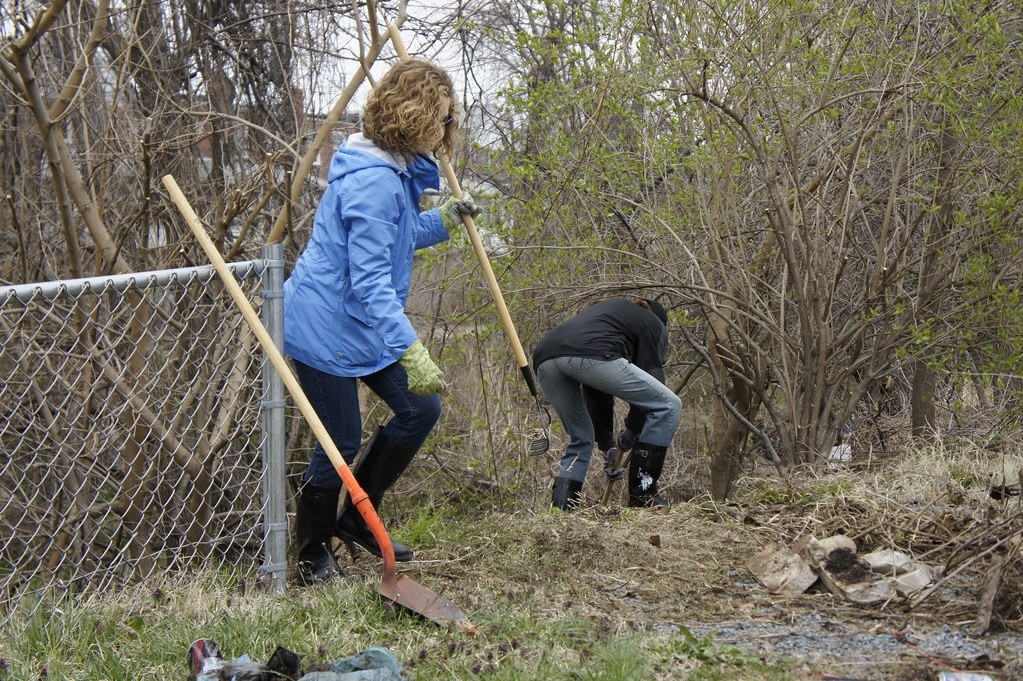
<box><xmin>399</xmin><ymin>339</ymin><xmax>447</xmax><ymax>396</ymax></box>
<box><xmin>600</xmin><ymin>448</ymin><xmax>625</xmax><ymax>481</ymax></box>
<box><xmin>617</xmin><ymin>428</ymin><xmax>637</xmax><ymax>451</ymax></box>
<box><xmin>435</xmin><ymin>192</ymin><xmax>483</xmax><ymax>230</ymax></box>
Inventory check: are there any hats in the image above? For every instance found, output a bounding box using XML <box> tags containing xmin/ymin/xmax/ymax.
<box><xmin>642</xmin><ymin>299</ymin><xmax>668</xmax><ymax>326</ymax></box>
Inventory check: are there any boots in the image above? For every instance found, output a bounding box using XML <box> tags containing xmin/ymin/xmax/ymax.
<box><xmin>552</xmin><ymin>477</ymin><xmax>583</xmax><ymax>511</ymax></box>
<box><xmin>297</xmin><ymin>471</ymin><xmax>346</xmax><ymax>587</ymax></box>
<box><xmin>628</xmin><ymin>439</ymin><xmax>669</xmax><ymax>509</ymax></box>
<box><xmin>334</xmin><ymin>425</ymin><xmax>418</xmax><ymax>561</ymax></box>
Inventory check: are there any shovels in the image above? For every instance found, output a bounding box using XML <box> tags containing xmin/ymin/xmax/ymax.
<box><xmin>160</xmin><ymin>170</ymin><xmax>474</xmax><ymax>630</ymax></box>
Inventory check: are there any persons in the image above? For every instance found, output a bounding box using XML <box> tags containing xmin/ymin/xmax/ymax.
<box><xmin>284</xmin><ymin>59</ymin><xmax>482</xmax><ymax>585</ymax></box>
<box><xmin>534</xmin><ymin>297</ymin><xmax>683</xmax><ymax>508</ymax></box>
